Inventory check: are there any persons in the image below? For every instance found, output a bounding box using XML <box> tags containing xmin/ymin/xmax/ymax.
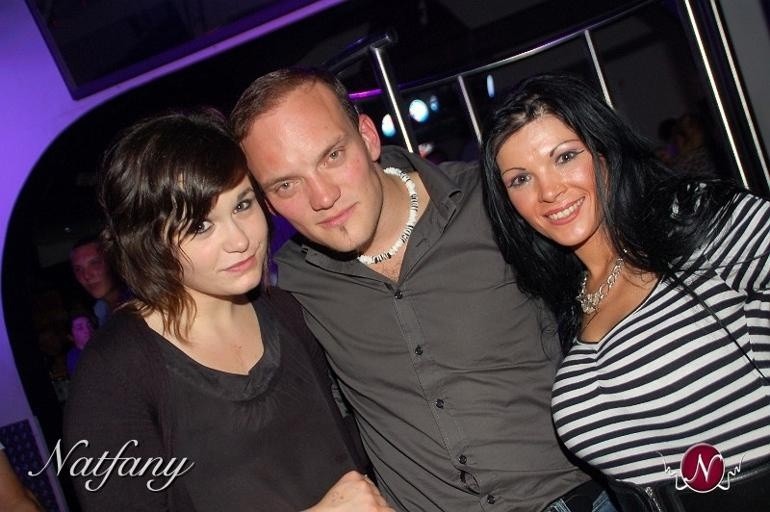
<box><xmin>657</xmin><ymin>116</ymin><xmax>727</xmax><ymax>179</ymax></box>
<box><xmin>65</xmin><ymin>313</ymin><xmax>96</xmax><ymax>378</ymax></box>
<box><xmin>63</xmin><ymin>113</ymin><xmax>399</xmax><ymax>511</ymax></box>
<box><xmin>480</xmin><ymin>72</ymin><xmax>770</xmax><ymax>512</ymax></box>
<box><xmin>232</xmin><ymin>66</ymin><xmax>623</xmax><ymax>512</ymax></box>
<box><xmin>67</xmin><ymin>238</ymin><xmax>133</xmax><ymax>326</ymax></box>
<box><xmin>0</xmin><ymin>444</ymin><xmax>45</xmax><ymax>512</ymax></box>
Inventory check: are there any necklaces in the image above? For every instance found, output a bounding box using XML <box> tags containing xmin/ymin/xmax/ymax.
<box><xmin>576</xmin><ymin>248</ymin><xmax>627</xmax><ymax>315</ymax></box>
<box><xmin>358</xmin><ymin>166</ymin><xmax>418</xmax><ymax>267</ymax></box>
